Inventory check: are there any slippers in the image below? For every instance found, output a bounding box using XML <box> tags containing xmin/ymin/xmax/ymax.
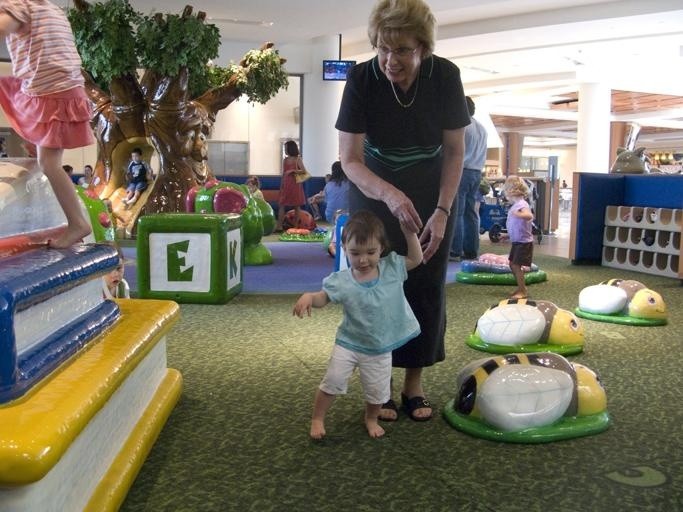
<box><xmin>402</xmin><ymin>392</ymin><xmax>432</xmax><ymax>422</ymax></box>
<box><xmin>377</xmin><ymin>400</ymin><xmax>399</xmax><ymax>422</ymax></box>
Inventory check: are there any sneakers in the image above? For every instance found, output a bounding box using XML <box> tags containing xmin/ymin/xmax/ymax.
<box><xmin>447</xmin><ymin>253</ymin><xmax>477</xmax><ymax>262</ymax></box>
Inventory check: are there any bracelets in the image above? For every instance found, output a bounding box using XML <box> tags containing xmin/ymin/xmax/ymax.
<box><xmin>437</xmin><ymin>206</ymin><xmax>452</xmax><ymax>217</ymax></box>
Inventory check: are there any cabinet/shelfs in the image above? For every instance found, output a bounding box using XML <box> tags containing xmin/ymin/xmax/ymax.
<box><xmin>600</xmin><ymin>204</ymin><xmax>683</xmax><ymax>280</ymax></box>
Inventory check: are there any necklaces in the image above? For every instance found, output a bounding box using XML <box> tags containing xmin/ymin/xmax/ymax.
<box><xmin>390</xmin><ymin>71</ymin><xmax>420</xmax><ymax>109</ymax></box>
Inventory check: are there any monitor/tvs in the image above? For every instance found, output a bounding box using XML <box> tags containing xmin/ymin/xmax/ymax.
<box><xmin>323</xmin><ymin>60</ymin><xmax>356</xmax><ymax>81</ymax></box>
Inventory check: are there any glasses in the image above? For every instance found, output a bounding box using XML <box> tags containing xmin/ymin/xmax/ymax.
<box><xmin>371</xmin><ymin>43</ymin><xmax>422</xmax><ymax>57</ymax></box>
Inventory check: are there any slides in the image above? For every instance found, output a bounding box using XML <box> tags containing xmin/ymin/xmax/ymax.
<box><xmin>96</xmin><ymin>135</ymin><xmax>163</xmax><ymax>238</ymax></box>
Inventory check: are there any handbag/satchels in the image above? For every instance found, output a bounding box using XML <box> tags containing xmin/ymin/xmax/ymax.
<box><xmin>294</xmin><ymin>172</ymin><xmax>311</xmax><ymax>184</ymax></box>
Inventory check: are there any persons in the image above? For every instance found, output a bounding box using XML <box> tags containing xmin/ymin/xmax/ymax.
<box><xmin>273</xmin><ymin>140</ymin><xmax>310</xmax><ymax>232</ymax></box>
<box><xmin>78</xmin><ymin>165</ymin><xmax>100</xmax><ymax>188</ymax></box>
<box><xmin>446</xmin><ymin>93</ymin><xmax>490</xmax><ymax>263</ymax></box>
<box><xmin>335</xmin><ymin>0</ymin><xmax>471</xmax><ymax>423</ymax></box>
<box><xmin>1</xmin><ymin>0</ymin><xmax>97</xmax><ymax>249</ymax></box>
<box><xmin>310</xmin><ymin>174</ymin><xmax>330</xmax><ymax>221</ymax></box>
<box><xmin>246</xmin><ymin>177</ymin><xmax>266</xmax><ymax>199</ymax></box>
<box><xmin>63</xmin><ymin>165</ymin><xmax>75</xmax><ymax>179</ymax></box>
<box><xmin>502</xmin><ymin>171</ymin><xmax>534</xmax><ymax>298</ymax></box>
<box><xmin>325</xmin><ymin>159</ymin><xmax>352</xmax><ymax>224</ymax></box>
<box><xmin>293</xmin><ymin>212</ymin><xmax>423</xmax><ymax>440</ymax></box>
<box><xmin>122</xmin><ymin>147</ymin><xmax>152</xmax><ymax>209</ymax></box>
